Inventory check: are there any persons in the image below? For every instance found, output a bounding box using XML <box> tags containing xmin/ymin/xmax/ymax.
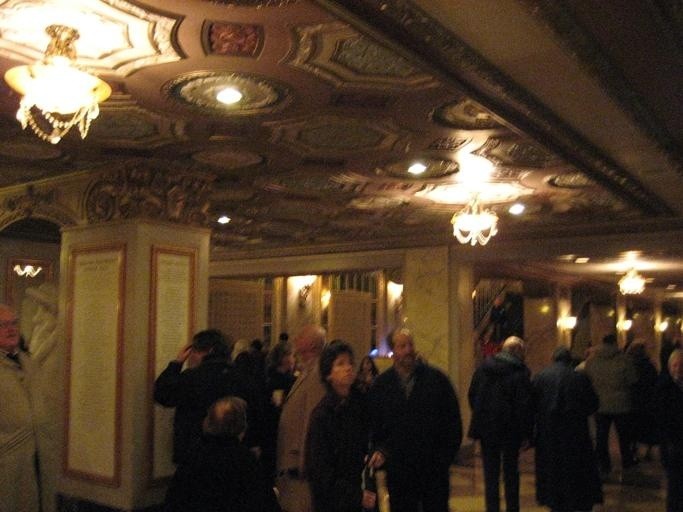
<box><xmin>299</xmin><ymin>339</ymin><xmax>371</xmax><ymax>512</ymax></box>
<box><xmin>150</xmin><ymin>322</ymin><xmax>326</xmax><ymax>512</ymax></box>
<box><xmin>357</xmin><ymin>326</ymin><xmax>464</xmax><ymax>512</ymax></box>
<box><xmin>569</xmin><ymin>330</ymin><xmax>682</xmax><ymax>512</ymax></box>
<box><xmin>466</xmin><ymin>334</ymin><xmax>531</xmax><ymax>512</ymax></box>
<box><xmin>528</xmin><ymin>347</ymin><xmax>601</xmax><ymax>512</ymax></box>
<box><xmin>0</xmin><ymin>300</ymin><xmax>59</xmax><ymax>512</ymax></box>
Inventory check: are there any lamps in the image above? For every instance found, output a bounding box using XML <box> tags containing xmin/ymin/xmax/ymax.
<box><xmin>2</xmin><ymin>25</ymin><xmax>111</xmax><ymax>144</ymax></box>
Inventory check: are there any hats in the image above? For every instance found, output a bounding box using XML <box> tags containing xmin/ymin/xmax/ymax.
<box><xmin>552</xmin><ymin>348</ymin><xmax>579</xmax><ymax>368</ymax></box>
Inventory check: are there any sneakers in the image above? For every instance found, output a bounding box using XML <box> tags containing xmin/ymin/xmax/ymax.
<box><xmin>623</xmin><ymin>456</ymin><xmax>638</xmax><ymax>466</ymax></box>
